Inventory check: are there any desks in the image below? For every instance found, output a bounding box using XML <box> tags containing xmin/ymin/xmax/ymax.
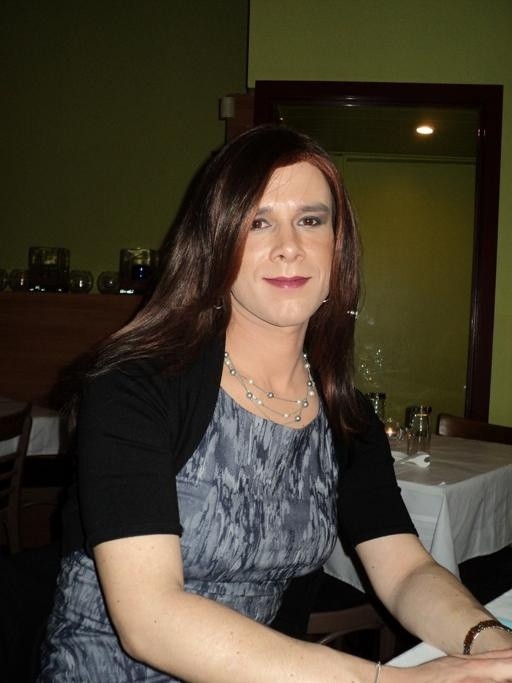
<box><xmin>0</xmin><ymin>397</ymin><xmax>62</xmax><ymax>458</ymax></box>
<box><xmin>0</xmin><ymin>291</ymin><xmax>142</xmax><ymax>410</ymax></box>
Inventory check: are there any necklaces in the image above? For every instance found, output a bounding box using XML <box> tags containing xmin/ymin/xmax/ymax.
<box><xmin>222</xmin><ymin>351</ymin><xmax>316</xmax><ymax>424</ymax></box>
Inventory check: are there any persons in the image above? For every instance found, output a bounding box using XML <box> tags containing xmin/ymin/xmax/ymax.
<box><xmin>6</xmin><ymin>125</ymin><xmax>511</xmax><ymax>683</ymax></box>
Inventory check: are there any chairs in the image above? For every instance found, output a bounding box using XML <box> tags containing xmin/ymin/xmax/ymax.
<box><xmin>0</xmin><ymin>402</ymin><xmax>33</xmax><ymax>557</ymax></box>
<box><xmin>277</xmin><ymin>564</ymin><xmax>397</xmax><ymax>668</ymax></box>
<box><xmin>436</xmin><ymin>412</ymin><xmax>512</xmax><ymax>446</ymax></box>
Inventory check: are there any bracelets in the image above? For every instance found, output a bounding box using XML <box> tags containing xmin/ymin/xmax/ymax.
<box><xmin>463</xmin><ymin>619</ymin><xmax>510</xmax><ymax>657</ymax></box>
<box><xmin>375</xmin><ymin>660</ymin><xmax>381</xmax><ymax>682</ymax></box>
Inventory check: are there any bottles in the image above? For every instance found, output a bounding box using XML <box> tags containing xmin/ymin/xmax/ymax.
<box><xmin>1</xmin><ymin>267</ymin><xmax>151</xmax><ymax>297</ymax></box>
<box><xmin>364</xmin><ymin>392</ymin><xmax>385</xmax><ymax>434</ymax></box>
<box><xmin>410</xmin><ymin>406</ymin><xmax>434</xmax><ymax>443</ymax></box>
<box><xmin>383</xmin><ymin>415</ymin><xmax>404</xmax><ymax>443</ymax></box>
